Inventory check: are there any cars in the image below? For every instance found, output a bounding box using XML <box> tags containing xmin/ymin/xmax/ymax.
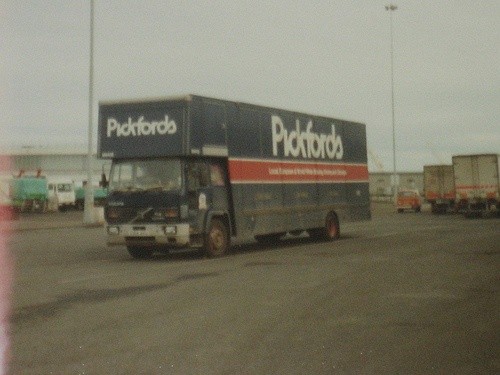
<box><xmin>394</xmin><ymin>189</ymin><xmax>421</xmax><ymax>213</ymax></box>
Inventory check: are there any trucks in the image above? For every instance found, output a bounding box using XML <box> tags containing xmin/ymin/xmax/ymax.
<box><xmin>46</xmin><ymin>177</ymin><xmax>76</xmax><ymax>211</ymax></box>
<box><xmin>93</xmin><ymin>95</ymin><xmax>373</xmax><ymax>256</ymax></box>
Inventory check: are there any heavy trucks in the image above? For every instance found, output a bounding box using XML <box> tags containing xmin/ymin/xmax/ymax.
<box><xmin>423</xmin><ymin>163</ymin><xmax>456</xmax><ymax>215</ymax></box>
<box><xmin>451</xmin><ymin>153</ymin><xmax>500</xmax><ymax>219</ymax></box>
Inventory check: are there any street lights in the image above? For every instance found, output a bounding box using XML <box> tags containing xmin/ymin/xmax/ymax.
<box><xmin>384</xmin><ymin>1</ymin><xmax>398</xmax><ymax>208</ymax></box>
